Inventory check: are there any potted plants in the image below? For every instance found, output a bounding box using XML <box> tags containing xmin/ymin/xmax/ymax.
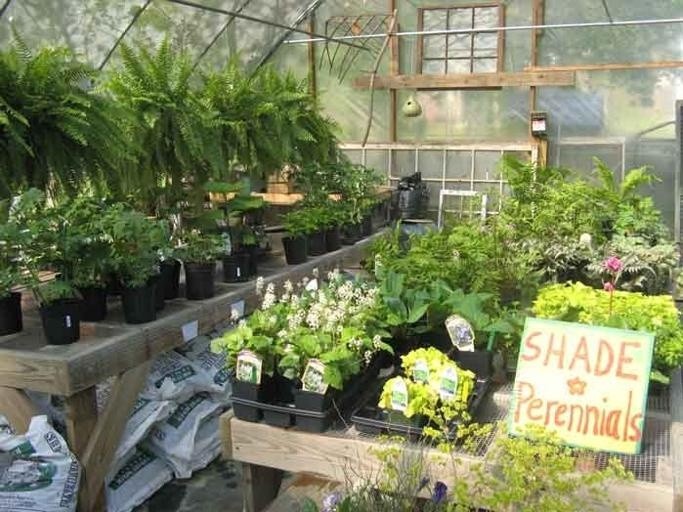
<box><xmin>277</xmin><ymin>163</ymin><xmax>387</xmax><ymax>266</ymax></box>
<box><xmin>1</xmin><ymin>176</ymin><xmax>271</xmax><ymax>344</ymax></box>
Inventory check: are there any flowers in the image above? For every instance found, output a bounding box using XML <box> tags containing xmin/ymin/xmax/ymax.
<box><xmin>216</xmin><ymin>264</ymin><xmax>387</xmax><ymax>390</ymax></box>
